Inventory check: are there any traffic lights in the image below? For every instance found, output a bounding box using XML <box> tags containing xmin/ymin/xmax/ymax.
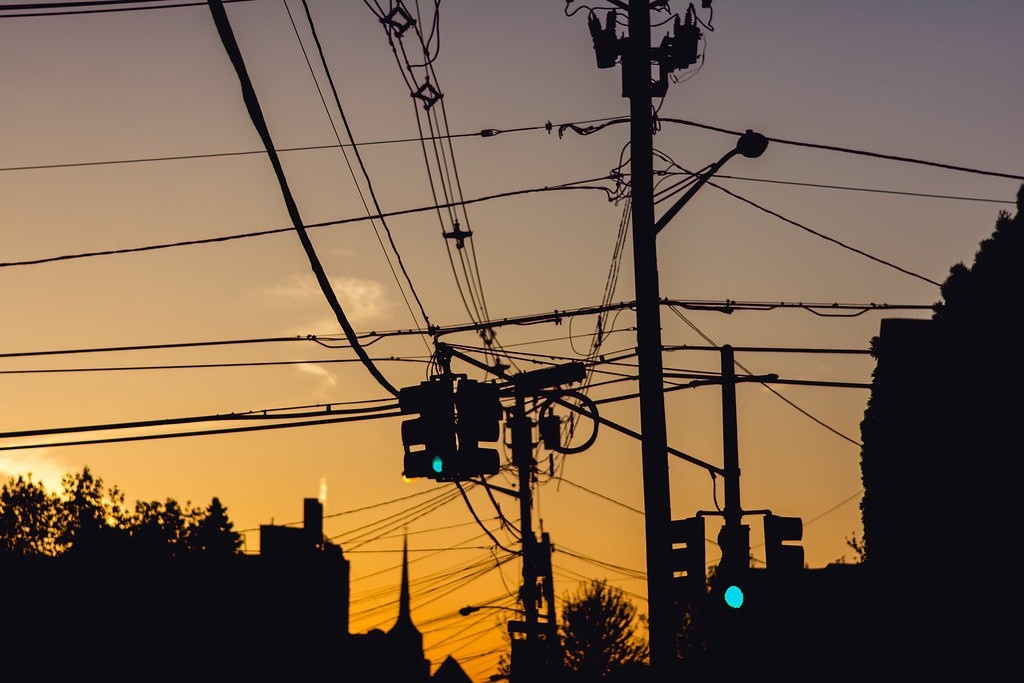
<box><xmin>459</xmin><ymin>378</ymin><xmax>503</xmax><ymax>476</ymax></box>
<box><xmin>419</xmin><ymin>376</ymin><xmax>454</xmax><ymax>485</ymax></box>
<box><xmin>764</xmin><ymin>513</ymin><xmax>806</xmax><ymax>596</ymax></box>
<box><xmin>667</xmin><ymin>516</ymin><xmax>707</xmax><ymax>604</ymax></box>
<box><xmin>716</xmin><ymin>524</ymin><xmax>749</xmax><ymax>616</ymax></box>
<box><xmin>399</xmin><ymin>378</ymin><xmax>431</xmax><ymax>479</ymax></box>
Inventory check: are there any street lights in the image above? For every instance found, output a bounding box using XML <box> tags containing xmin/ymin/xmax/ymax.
<box><xmin>621</xmin><ymin>118</ymin><xmax>775</xmax><ymax>683</ymax></box>
<box><xmin>458</xmin><ymin>604</ymin><xmax>565</xmax><ymax>683</ymax></box>
